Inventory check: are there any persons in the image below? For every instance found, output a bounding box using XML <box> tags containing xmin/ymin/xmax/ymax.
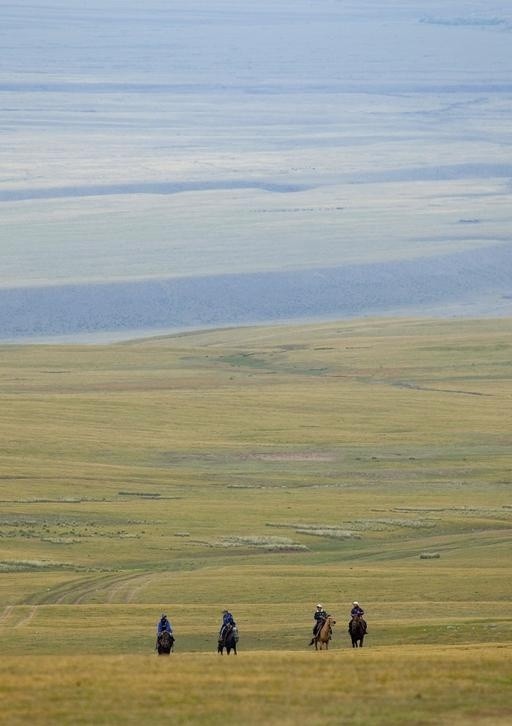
<box><xmin>348</xmin><ymin>601</ymin><xmax>368</xmax><ymax>634</ymax></box>
<box><xmin>313</xmin><ymin>604</ymin><xmax>334</xmax><ymax>640</ymax></box>
<box><xmin>156</xmin><ymin>613</ymin><xmax>176</xmax><ymax>652</ymax></box>
<box><xmin>219</xmin><ymin>609</ymin><xmax>238</xmax><ymax>643</ymax></box>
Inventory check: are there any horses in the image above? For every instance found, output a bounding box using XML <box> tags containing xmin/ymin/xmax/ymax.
<box><xmin>157</xmin><ymin>632</ymin><xmax>174</xmax><ymax>655</ymax></box>
<box><xmin>218</xmin><ymin>627</ymin><xmax>240</xmax><ymax>655</ymax></box>
<box><xmin>314</xmin><ymin>615</ymin><xmax>336</xmax><ymax>650</ymax></box>
<box><xmin>348</xmin><ymin>613</ymin><xmax>367</xmax><ymax>648</ymax></box>
